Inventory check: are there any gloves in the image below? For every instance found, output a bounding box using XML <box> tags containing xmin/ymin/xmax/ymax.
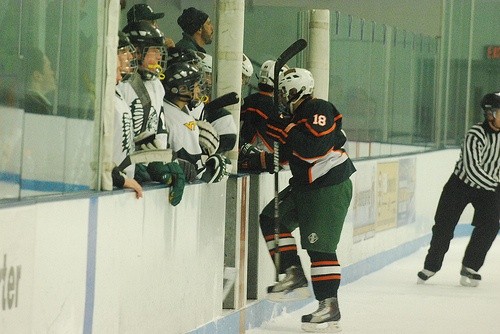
<box><xmin>134</xmin><ymin>160</ymin><xmax>186</xmax><ymax>207</ymax></box>
<box><xmin>197</xmin><ymin>120</ymin><xmax>221</xmax><ymax>155</ymax></box>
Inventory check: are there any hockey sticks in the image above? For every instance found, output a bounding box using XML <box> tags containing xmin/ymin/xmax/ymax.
<box><xmin>272</xmin><ymin>37</ymin><xmax>308</xmax><ymax>284</ymax></box>
<box><xmin>204</xmin><ymin>91</ymin><xmax>240</xmax><ymax>124</ymax></box>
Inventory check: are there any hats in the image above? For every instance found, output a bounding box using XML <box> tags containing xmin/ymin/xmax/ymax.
<box><xmin>177</xmin><ymin>7</ymin><xmax>209</xmax><ymax>35</ymax></box>
<box><xmin>127</xmin><ymin>4</ymin><xmax>165</xmax><ymax>21</ymax></box>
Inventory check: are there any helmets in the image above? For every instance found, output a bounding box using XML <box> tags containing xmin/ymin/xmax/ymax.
<box><xmin>162</xmin><ymin>62</ymin><xmax>205</xmax><ymax>101</ymax></box>
<box><xmin>121</xmin><ymin>21</ymin><xmax>165</xmax><ymax>79</ymax></box>
<box><xmin>279</xmin><ymin>67</ymin><xmax>314</xmax><ymax>112</ymax></box>
<box><xmin>482</xmin><ymin>92</ymin><xmax>500</xmax><ymax>108</ymax></box>
<box><xmin>195</xmin><ymin>51</ymin><xmax>213</xmax><ymax>88</ymax></box>
<box><xmin>242</xmin><ymin>53</ymin><xmax>254</xmax><ymax>77</ymax></box>
<box><xmin>164</xmin><ymin>47</ymin><xmax>200</xmax><ymax>70</ymax></box>
<box><xmin>258</xmin><ymin>59</ymin><xmax>290</xmax><ymax>92</ymax></box>
<box><xmin>117</xmin><ymin>31</ymin><xmax>138</xmax><ymax>74</ymax></box>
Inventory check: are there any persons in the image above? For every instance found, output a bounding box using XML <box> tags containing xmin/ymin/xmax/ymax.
<box><xmin>100</xmin><ymin>3</ymin><xmax>253</xmax><ymax>206</ymax></box>
<box><xmin>238</xmin><ymin>60</ymin><xmax>290</xmax><ymax>174</ymax></box>
<box><xmin>259</xmin><ymin>66</ymin><xmax>357</xmax><ymax>332</ymax></box>
<box><xmin>418</xmin><ymin>92</ymin><xmax>500</xmax><ymax>287</ymax></box>
<box><xmin>18</xmin><ymin>47</ymin><xmax>57</xmax><ymax>116</ymax></box>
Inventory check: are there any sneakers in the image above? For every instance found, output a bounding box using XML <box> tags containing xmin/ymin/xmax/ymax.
<box><xmin>301</xmin><ymin>297</ymin><xmax>342</xmax><ymax>333</ymax></box>
<box><xmin>460</xmin><ymin>266</ymin><xmax>481</xmax><ymax>286</ymax></box>
<box><xmin>266</xmin><ymin>266</ymin><xmax>309</xmax><ymax>301</ymax></box>
<box><xmin>416</xmin><ymin>269</ymin><xmax>435</xmax><ymax>285</ymax></box>
